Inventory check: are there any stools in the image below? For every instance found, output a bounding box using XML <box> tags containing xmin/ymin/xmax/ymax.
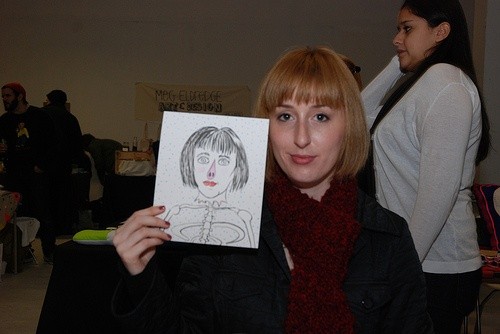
<box><xmin>16</xmin><ymin>215</ymin><xmax>40</xmax><ymax>265</ymax></box>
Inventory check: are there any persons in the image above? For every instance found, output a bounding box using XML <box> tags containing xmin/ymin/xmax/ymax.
<box><xmin>360</xmin><ymin>0</ymin><xmax>490</xmax><ymax>334</ymax></box>
<box><xmin>111</xmin><ymin>46</ymin><xmax>434</xmax><ymax>334</ymax></box>
<box><xmin>0</xmin><ymin>83</ymin><xmax>123</xmax><ymax>265</ymax></box>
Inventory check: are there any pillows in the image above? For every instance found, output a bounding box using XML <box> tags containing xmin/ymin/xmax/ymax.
<box><xmin>474</xmin><ymin>184</ymin><xmax>500</xmax><ymax>251</ymax></box>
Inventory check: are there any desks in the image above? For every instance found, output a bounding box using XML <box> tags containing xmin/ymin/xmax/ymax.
<box><xmin>35</xmin><ymin>240</ymin><xmax>124</xmax><ymax>334</ymax></box>
<box><xmin>100</xmin><ymin>175</ymin><xmax>155</xmax><ymax>228</ymax></box>
<box><xmin>0</xmin><ymin>189</ymin><xmax>20</xmax><ymax>276</ymax></box>
